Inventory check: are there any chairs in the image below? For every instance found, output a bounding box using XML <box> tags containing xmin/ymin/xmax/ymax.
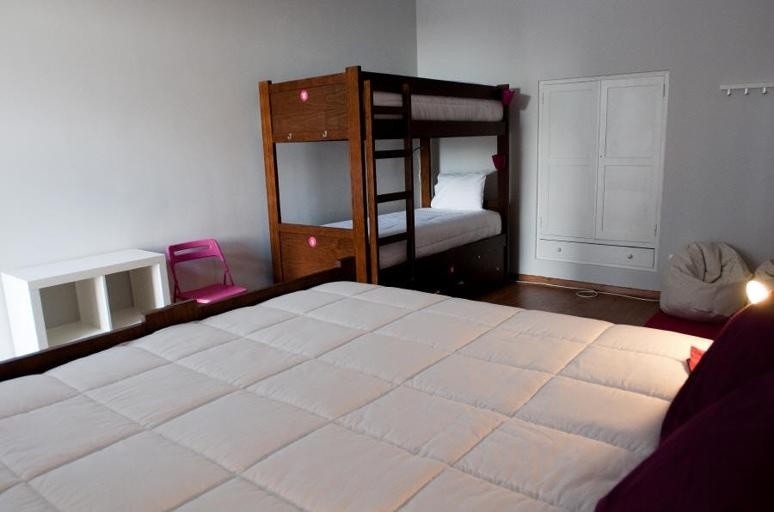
<box><xmin>164</xmin><ymin>237</ymin><xmax>246</xmax><ymax>306</ymax></box>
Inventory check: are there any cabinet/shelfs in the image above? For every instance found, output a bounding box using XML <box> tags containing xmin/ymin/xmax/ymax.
<box><xmin>2</xmin><ymin>247</ymin><xmax>171</xmax><ymax>356</ymax></box>
<box><xmin>536</xmin><ymin>72</ymin><xmax>669</xmax><ymax>274</ymax></box>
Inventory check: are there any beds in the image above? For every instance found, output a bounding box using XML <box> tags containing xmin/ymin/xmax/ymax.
<box><xmin>258</xmin><ymin>64</ymin><xmax>512</xmax><ymax>294</ymax></box>
<box><xmin>0</xmin><ymin>258</ymin><xmax>774</xmax><ymax>511</ymax></box>
